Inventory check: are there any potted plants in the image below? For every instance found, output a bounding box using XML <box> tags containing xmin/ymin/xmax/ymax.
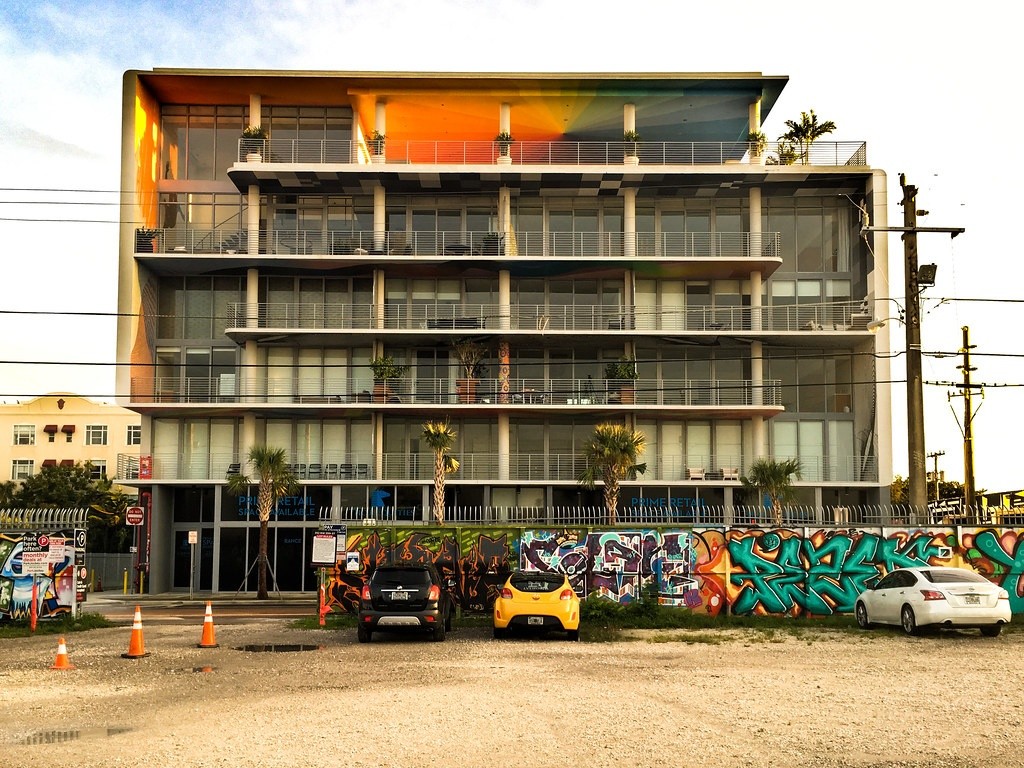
<box><xmin>137</xmin><ymin>227</ymin><xmax>159</xmax><ymax>252</ymax></box>
<box><xmin>365</xmin><ymin>356</ymin><xmax>409</xmax><ymax>403</ymax></box>
<box><xmin>453</xmin><ymin>338</ymin><xmax>487</xmax><ymax>403</ymax></box>
<box><xmin>492</xmin><ymin>132</ymin><xmax>515</xmax><ymax>166</ymax></box>
<box><xmin>367</xmin><ymin>131</ymin><xmax>388</xmax><ymax>163</ymax></box>
<box><xmin>607</xmin><ymin>356</ymin><xmax>641</xmax><ymax>405</ymax></box>
<box><xmin>623</xmin><ymin>130</ymin><xmax>642</xmax><ymax>165</ymax></box>
<box><xmin>241</xmin><ymin>127</ymin><xmax>268</xmax><ymax>163</ymax></box>
<box><xmin>745</xmin><ymin>129</ymin><xmax>765</xmax><ymax>163</ymax></box>
<box><xmin>483</xmin><ymin>232</ymin><xmax>505</xmax><ymax>255</ymax></box>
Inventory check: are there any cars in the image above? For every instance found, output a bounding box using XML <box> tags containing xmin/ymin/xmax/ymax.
<box><xmin>855</xmin><ymin>567</ymin><xmax>1012</xmax><ymax>638</ymax></box>
<box><xmin>494</xmin><ymin>570</ymin><xmax>584</xmax><ymax>642</ymax></box>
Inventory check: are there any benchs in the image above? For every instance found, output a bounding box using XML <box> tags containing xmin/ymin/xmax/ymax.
<box><xmin>426</xmin><ymin>317</ymin><xmax>487</xmax><ymax>329</ymax></box>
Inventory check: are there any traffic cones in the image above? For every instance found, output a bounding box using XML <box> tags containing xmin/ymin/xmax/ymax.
<box><xmin>50</xmin><ymin>638</ymin><xmax>74</xmax><ymax>669</ymax></box>
<box><xmin>199</xmin><ymin>602</ymin><xmax>219</xmax><ymax>648</ymax></box>
<box><xmin>120</xmin><ymin>605</ymin><xmax>152</xmax><ymax>660</ymax></box>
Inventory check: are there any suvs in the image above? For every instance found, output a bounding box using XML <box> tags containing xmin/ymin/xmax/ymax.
<box><xmin>357</xmin><ymin>561</ymin><xmax>457</xmax><ymax>642</ymax></box>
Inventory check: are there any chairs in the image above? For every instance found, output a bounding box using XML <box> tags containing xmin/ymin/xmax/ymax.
<box><xmin>687</xmin><ymin>468</ymin><xmax>706</xmax><ymax>481</ymax></box>
<box><xmin>340</xmin><ymin>464</ymin><xmax>352</xmax><ymax>479</ymax></box>
<box><xmin>389</xmin><ymin>394</ymin><xmax>402</xmax><ymax>404</ymax></box>
<box><xmin>325</xmin><ymin>464</ymin><xmax>337</xmax><ymax>479</ymax></box>
<box><xmin>720</xmin><ymin>468</ymin><xmax>739</xmax><ymax>480</ymax></box>
<box><xmin>294</xmin><ymin>464</ymin><xmax>307</xmax><ymax>479</ymax></box>
<box><xmin>226</xmin><ymin>464</ymin><xmax>240</xmax><ymax>479</ymax></box>
<box><xmin>281</xmin><ymin>464</ymin><xmax>292</xmax><ymax>476</ymax></box>
<box><xmin>364</xmin><ymin>391</ymin><xmax>375</xmax><ymax>403</ymax></box>
<box><xmin>512</xmin><ymin>394</ymin><xmax>523</xmax><ymax>404</ymax></box>
<box><xmin>355</xmin><ymin>464</ymin><xmax>368</xmax><ymax>479</ymax></box>
<box><xmin>309</xmin><ymin>464</ymin><xmax>322</xmax><ymax>478</ymax></box>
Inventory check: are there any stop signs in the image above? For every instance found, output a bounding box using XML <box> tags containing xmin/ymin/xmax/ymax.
<box><xmin>125</xmin><ymin>507</ymin><xmax>144</xmax><ymax>525</ymax></box>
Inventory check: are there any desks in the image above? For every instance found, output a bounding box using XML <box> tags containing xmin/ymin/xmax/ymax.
<box><xmin>705</xmin><ymin>472</ymin><xmax>724</xmax><ymax>479</ymax></box>
